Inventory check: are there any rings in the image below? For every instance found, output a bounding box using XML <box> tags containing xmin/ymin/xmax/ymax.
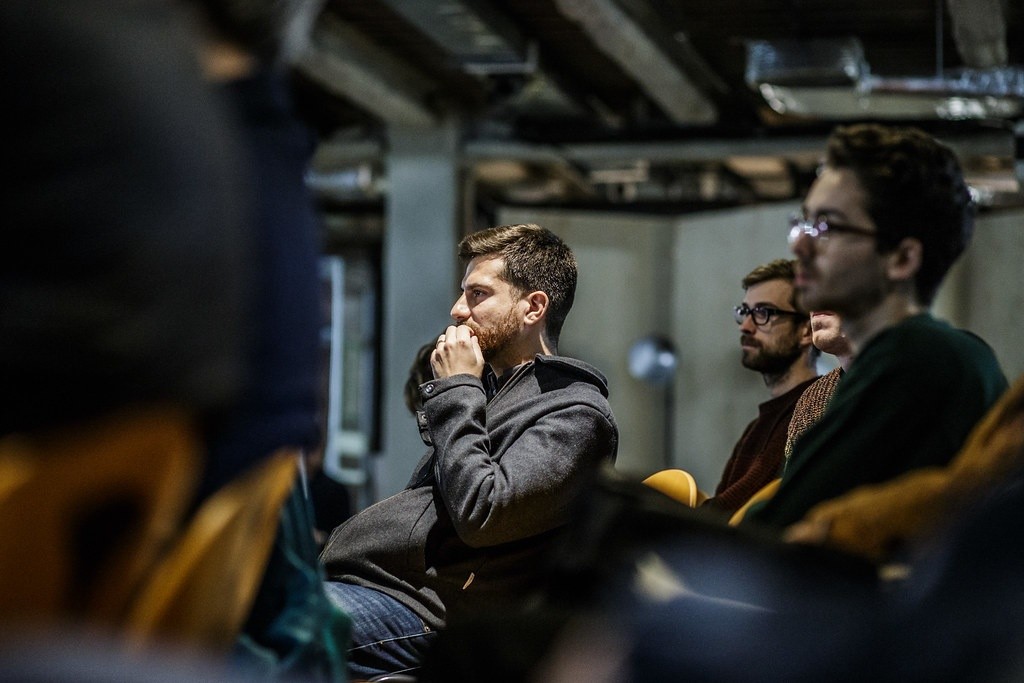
<box><xmin>435</xmin><ymin>340</ymin><xmax>443</xmax><ymax>350</ymax></box>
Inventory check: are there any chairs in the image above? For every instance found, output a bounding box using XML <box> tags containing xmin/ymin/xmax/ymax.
<box><xmin>1</xmin><ymin>416</ymin><xmax>359</xmax><ymax>683</ymax></box>
<box><xmin>641</xmin><ymin>468</ymin><xmax>697</xmax><ymax>508</ymax></box>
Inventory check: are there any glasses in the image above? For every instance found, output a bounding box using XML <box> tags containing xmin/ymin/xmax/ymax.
<box><xmin>789</xmin><ymin>210</ymin><xmax>876</xmax><ymax>238</ymax></box>
<box><xmin>734</xmin><ymin>305</ymin><xmax>803</xmax><ymax>326</ymax></box>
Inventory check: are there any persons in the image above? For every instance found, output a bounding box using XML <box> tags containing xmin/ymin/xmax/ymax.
<box><xmin>311</xmin><ymin>222</ymin><xmax>618</xmax><ymax>683</ymax></box>
<box><xmin>727</xmin><ymin>123</ymin><xmax>1010</xmax><ymax>548</ymax></box>
<box><xmin>702</xmin><ymin>253</ymin><xmax>858</xmax><ymax>520</ymax></box>
